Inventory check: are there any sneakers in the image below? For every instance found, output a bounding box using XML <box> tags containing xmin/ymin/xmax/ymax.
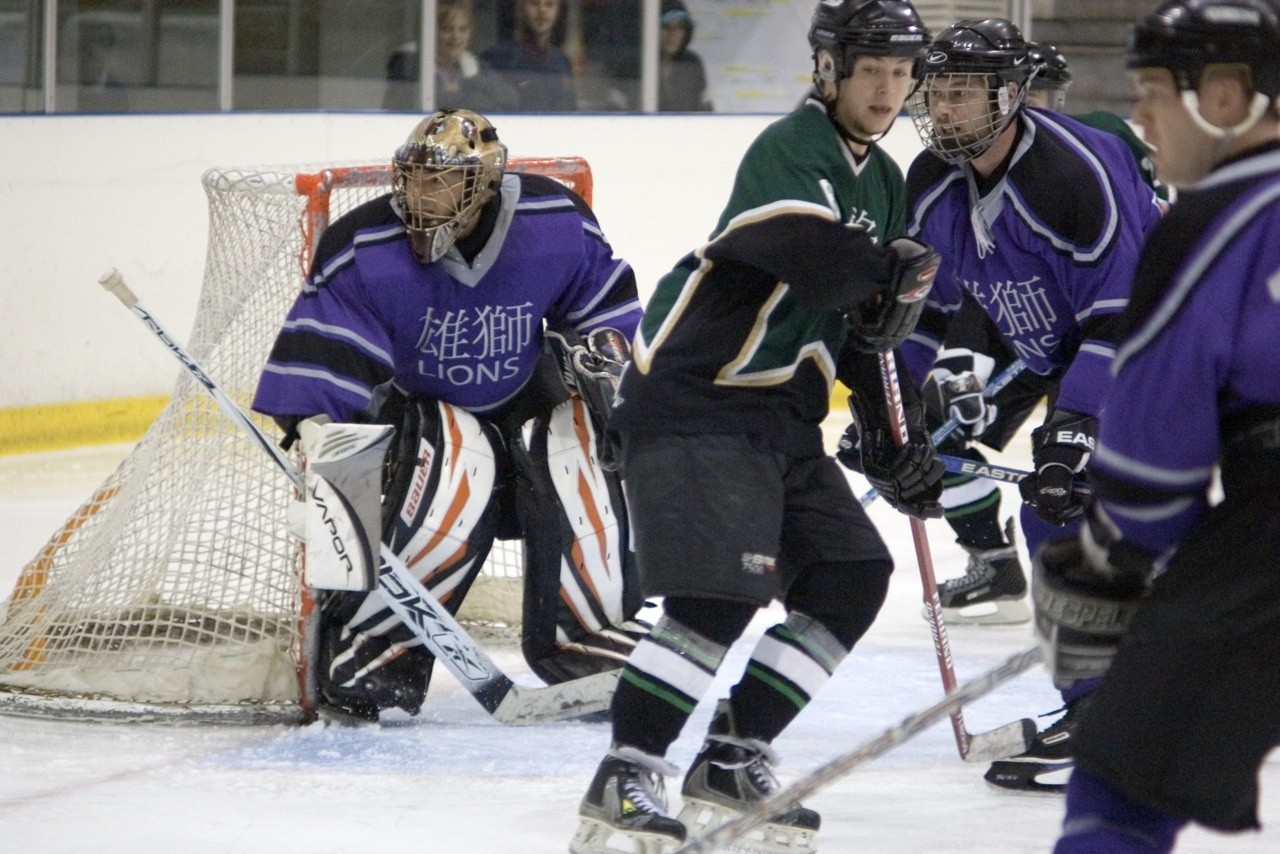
<box><xmin>566</xmin><ymin>746</ymin><xmax>688</xmax><ymax>854</ymax></box>
<box><xmin>675</xmin><ymin>696</ymin><xmax>820</xmax><ymax>854</ymax></box>
<box><xmin>921</xmin><ymin>516</ymin><xmax>1028</xmax><ymax>625</ymax></box>
<box><xmin>985</xmin><ymin>693</ymin><xmax>1076</xmax><ymax>789</ymax></box>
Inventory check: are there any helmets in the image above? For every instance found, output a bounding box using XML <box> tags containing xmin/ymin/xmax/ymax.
<box><xmin>805</xmin><ymin>1</ymin><xmax>933</xmax><ymax>79</ymax></box>
<box><xmin>907</xmin><ymin>13</ymin><xmax>1030</xmax><ymax>160</ymax></box>
<box><xmin>394</xmin><ymin>105</ymin><xmax>505</xmax><ymax>251</ymax></box>
<box><xmin>1023</xmin><ymin>40</ymin><xmax>1075</xmax><ymax>115</ymax></box>
<box><xmin>1122</xmin><ymin>0</ymin><xmax>1280</xmax><ymax>97</ymax></box>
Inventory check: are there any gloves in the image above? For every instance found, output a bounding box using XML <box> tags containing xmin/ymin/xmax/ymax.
<box><xmin>1016</xmin><ymin>411</ymin><xmax>1098</xmax><ymax>522</ymax></box>
<box><xmin>837</xmin><ymin>369</ymin><xmax>969</xmax><ymax>472</ymax></box>
<box><xmin>843</xmin><ymin>240</ymin><xmax>938</xmax><ymax>352</ymax></box>
<box><xmin>847</xmin><ymin>395</ymin><xmax>946</xmax><ymax>519</ymax></box>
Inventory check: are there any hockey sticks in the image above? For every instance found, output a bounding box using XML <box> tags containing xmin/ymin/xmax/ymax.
<box><xmin>96</xmin><ymin>267</ymin><xmax>624</xmax><ymax>729</ymax></box>
<box><xmin>928</xmin><ymin>449</ymin><xmax>1034</xmax><ymax>485</ymax></box>
<box><xmin>677</xmin><ymin>648</ymin><xmax>1044</xmax><ymax>854</ymax></box>
<box><xmin>857</xmin><ymin>359</ymin><xmax>1026</xmax><ymax>513</ymax></box>
<box><xmin>878</xmin><ymin>345</ymin><xmax>1037</xmax><ymax>763</ymax></box>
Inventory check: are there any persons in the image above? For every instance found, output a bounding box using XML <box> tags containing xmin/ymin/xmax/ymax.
<box><xmin>841</xmin><ymin>0</ymin><xmax>1280</xmax><ymax>854</ymax></box>
<box><xmin>383</xmin><ymin>0</ymin><xmax>714</xmax><ymax>114</ymax></box>
<box><xmin>247</xmin><ymin>110</ymin><xmax>650</xmax><ymax>725</ymax></box>
<box><xmin>572</xmin><ymin>0</ymin><xmax>942</xmax><ymax>854</ymax></box>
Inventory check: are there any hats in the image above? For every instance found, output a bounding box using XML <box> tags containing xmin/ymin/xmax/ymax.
<box><xmin>658</xmin><ymin>8</ymin><xmax>684</xmax><ymax>24</ymax></box>
